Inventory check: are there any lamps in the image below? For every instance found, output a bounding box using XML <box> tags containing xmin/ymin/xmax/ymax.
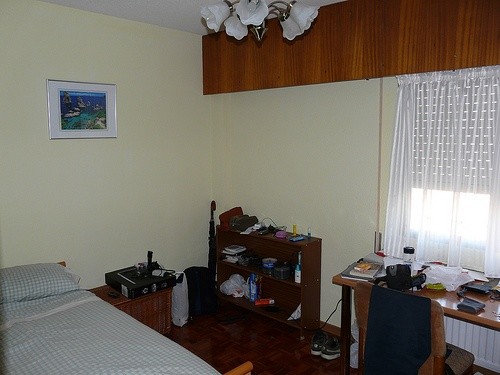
<box><xmin>200</xmin><ymin>0</ymin><xmax>320</xmax><ymax>42</ymax></box>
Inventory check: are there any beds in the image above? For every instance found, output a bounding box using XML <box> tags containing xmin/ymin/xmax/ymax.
<box><xmin>0</xmin><ymin>261</ymin><xmax>253</xmax><ymax>375</ymax></box>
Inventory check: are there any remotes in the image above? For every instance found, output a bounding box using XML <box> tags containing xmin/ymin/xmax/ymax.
<box><xmin>107</xmin><ymin>291</ymin><xmax>120</xmax><ymax>298</ymax></box>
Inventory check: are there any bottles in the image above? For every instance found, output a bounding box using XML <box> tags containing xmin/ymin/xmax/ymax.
<box><xmin>295</xmin><ymin>265</ymin><xmax>301</xmax><ymax>283</ymax></box>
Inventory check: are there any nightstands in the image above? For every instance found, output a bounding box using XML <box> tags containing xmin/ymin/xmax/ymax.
<box><xmin>87</xmin><ymin>285</ymin><xmax>172</xmax><ymax>335</ymax></box>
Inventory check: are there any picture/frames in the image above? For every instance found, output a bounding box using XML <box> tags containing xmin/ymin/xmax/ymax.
<box><xmin>46</xmin><ymin>78</ymin><xmax>117</xmax><ymax>140</ymax></box>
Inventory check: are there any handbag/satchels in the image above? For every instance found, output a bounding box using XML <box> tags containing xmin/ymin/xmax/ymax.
<box><xmin>374</xmin><ymin>264</ymin><xmax>413</xmax><ymax>293</ymax></box>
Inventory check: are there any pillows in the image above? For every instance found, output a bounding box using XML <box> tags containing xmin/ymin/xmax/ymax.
<box><xmin>0</xmin><ymin>263</ymin><xmax>80</xmax><ymax>304</ymax></box>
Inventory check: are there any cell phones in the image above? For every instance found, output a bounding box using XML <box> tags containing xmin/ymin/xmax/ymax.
<box><xmin>290</xmin><ymin>236</ymin><xmax>305</xmax><ymax>242</ymax></box>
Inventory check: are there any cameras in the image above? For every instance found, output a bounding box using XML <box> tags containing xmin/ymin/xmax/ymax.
<box><xmin>375</xmin><ymin>264</ymin><xmax>426</xmax><ymax>291</ymax></box>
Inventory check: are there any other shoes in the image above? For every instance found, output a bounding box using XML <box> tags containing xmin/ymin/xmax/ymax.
<box><xmin>320</xmin><ymin>334</ymin><xmax>340</xmax><ymax>360</ymax></box>
<box><xmin>310</xmin><ymin>331</ymin><xmax>328</xmax><ymax>356</ymax></box>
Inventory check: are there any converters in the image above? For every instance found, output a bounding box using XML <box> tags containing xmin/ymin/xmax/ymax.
<box><xmin>266</xmin><ymin>306</ymin><xmax>280</xmax><ymax>313</ymax></box>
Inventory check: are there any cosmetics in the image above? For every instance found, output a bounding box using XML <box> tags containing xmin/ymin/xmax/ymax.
<box><xmin>249</xmin><ymin>222</ymin><xmax>311</xmax><ymax>305</ymax></box>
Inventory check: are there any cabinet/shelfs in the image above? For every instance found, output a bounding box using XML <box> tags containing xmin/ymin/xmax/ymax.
<box><xmin>216</xmin><ymin>225</ymin><xmax>322</xmax><ymax>341</ymax></box>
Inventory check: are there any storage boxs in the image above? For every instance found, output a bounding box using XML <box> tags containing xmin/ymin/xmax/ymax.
<box><xmin>221</xmin><ymin>245</ymin><xmax>246</xmax><ymax>262</ymax></box>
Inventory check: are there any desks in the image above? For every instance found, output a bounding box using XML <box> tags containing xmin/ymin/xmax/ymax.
<box><xmin>332</xmin><ymin>255</ymin><xmax>500</xmax><ymax>375</ymax></box>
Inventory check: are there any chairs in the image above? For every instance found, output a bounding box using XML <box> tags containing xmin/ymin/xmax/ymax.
<box><xmin>354</xmin><ymin>279</ymin><xmax>474</xmax><ymax>375</ymax></box>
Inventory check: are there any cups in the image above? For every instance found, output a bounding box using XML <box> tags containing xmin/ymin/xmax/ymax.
<box><xmin>403</xmin><ymin>247</ymin><xmax>414</xmax><ymax>276</ymax></box>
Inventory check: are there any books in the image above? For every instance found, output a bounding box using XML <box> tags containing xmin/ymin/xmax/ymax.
<box><xmin>340</xmin><ymin>261</ymin><xmax>382</xmax><ymax>282</ymax></box>
